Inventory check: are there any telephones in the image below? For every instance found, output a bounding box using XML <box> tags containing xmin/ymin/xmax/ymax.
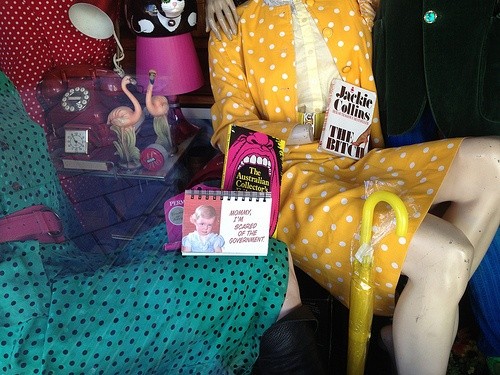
<box><xmin>37</xmin><ymin>65</ymin><xmax>125</xmax><ymax>146</ymax></box>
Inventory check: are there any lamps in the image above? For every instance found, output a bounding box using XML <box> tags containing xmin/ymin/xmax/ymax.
<box><xmin>67</xmin><ymin>0</ymin><xmax>126</xmax><ymax>77</ymax></box>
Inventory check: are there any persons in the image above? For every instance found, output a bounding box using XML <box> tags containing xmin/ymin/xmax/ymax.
<box><xmin>207</xmin><ymin>0</ymin><xmax>500</xmax><ymax>374</ymax></box>
<box><xmin>351</xmin><ymin>124</ymin><xmax>372</xmax><ymax>150</ymax></box>
<box><xmin>372</xmin><ymin>0</ymin><xmax>500</xmax><ymax>374</ymax></box>
<box><xmin>184</xmin><ymin>205</ymin><xmax>226</xmax><ymax>253</ymax></box>
<box><xmin>2</xmin><ymin>75</ymin><xmax>331</xmax><ymax>375</ymax></box>
<box><xmin>206</xmin><ymin>0</ymin><xmax>242</xmax><ymax>42</ymax></box>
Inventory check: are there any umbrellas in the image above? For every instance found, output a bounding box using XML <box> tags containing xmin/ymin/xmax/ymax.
<box><xmin>347</xmin><ymin>190</ymin><xmax>410</xmax><ymax>373</ymax></box>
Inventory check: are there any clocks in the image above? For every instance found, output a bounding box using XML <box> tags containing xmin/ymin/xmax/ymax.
<box><xmin>62</xmin><ymin>124</ymin><xmax>103</xmax><ymax>161</ymax></box>
